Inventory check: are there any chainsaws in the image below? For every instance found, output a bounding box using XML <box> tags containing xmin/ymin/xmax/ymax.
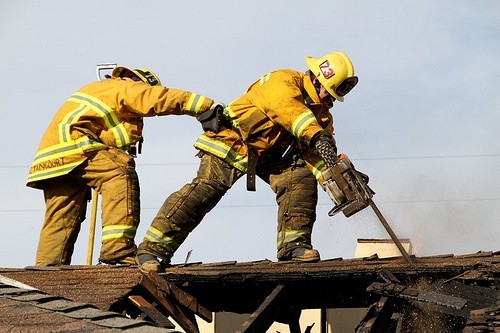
<box><xmin>311</xmin><ymin>153</ymin><xmax>413</xmax><ymax>264</ymax></box>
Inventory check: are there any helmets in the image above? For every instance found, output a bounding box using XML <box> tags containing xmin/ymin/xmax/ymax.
<box><xmin>306</xmin><ymin>51</ymin><xmax>359</xmax><ymax>102</ymax></box>
<box><xmin>112</xmin><ymin>67</ymin><xmax>161</xmax><ymax>86</ymax></box>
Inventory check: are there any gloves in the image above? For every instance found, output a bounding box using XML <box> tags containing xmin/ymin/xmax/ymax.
<box><xmin>195</xmin><ymin>102</ymin><xmax>226</xmax><ymax>135</ymax></box>
<box><xmin>313</xmin><ymin>130</ymin><xmax>337</xmax><ymax>158</ymax></box>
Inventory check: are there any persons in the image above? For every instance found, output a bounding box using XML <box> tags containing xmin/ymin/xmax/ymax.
<box><xmin>27</xmin><ymin>67</ymin><xmax>234</xmax><ymax>267</ymax></box>
<box><xmin>134</xmin><ymin>51</ymin><xmax>375</xmax><ymax>274</ymax></box>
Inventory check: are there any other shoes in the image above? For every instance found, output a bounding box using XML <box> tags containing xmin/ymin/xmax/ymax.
<box><xmin>101</xmin><ymin>253</ymin><xmax>136</xmax><ymax>264</ymax></box>
<box><xmin>278</xmin><ymin>248</ymin><xmax>321</xmax><ymax>261</ymax></box>
<box><xmin>135</xmin><ymin>253</ymin><xmax>161</xmax><ymax>273</ymax></box>
